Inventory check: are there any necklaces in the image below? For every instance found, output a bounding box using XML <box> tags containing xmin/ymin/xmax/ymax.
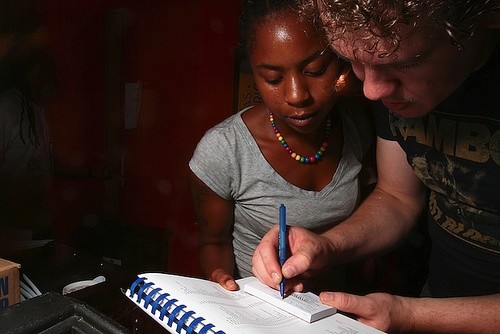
<box><xmin>270</xmin><ymin>109</ymin><xmax>332</xmax><ymax>163</ymax></box>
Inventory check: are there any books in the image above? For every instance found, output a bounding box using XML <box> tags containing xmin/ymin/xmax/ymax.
<box><xmin>125</xmin><ymin>272</ymin><xmax>388</xmax><ymax>334</ymax></box>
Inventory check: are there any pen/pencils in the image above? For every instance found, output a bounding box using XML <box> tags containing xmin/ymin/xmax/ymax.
<box><xmin>279</xmin><ymin>204</ymin><xmax>287</xmax><ymax>300</ymax></box>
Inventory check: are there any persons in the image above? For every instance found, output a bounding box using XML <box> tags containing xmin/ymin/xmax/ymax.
<box><xmin>252</xmin><ymin>0</ymin><xmax>500</xmax><ymax>334</ymax></box>
<box><xmin>188</xmin><ymin>0</ymin><xmax>363</xmax><ymax>292</ymax></box>
